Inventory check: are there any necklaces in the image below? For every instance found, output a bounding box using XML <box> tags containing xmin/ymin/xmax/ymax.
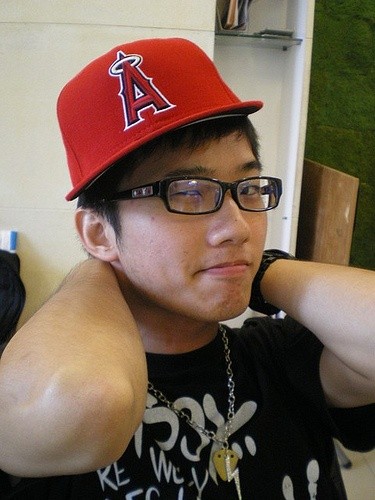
<box><xmin>148</xmin><ymin>323</ymin><xmax>244</xmax><ymax>500</ymax></box>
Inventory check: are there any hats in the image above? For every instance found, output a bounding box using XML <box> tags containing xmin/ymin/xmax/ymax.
<box><xmin>57</xmin><ymin>38</ymin><xmax>263</xmax><ymax>201</ymax></box>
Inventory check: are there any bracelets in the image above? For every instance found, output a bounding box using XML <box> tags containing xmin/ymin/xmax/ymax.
<box><xmin>249</xmin><ymin>248</ymin><xmax>296</xmax><ymax>315</ymax></box>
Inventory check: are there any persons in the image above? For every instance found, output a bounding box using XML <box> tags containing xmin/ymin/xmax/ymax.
<box><xmin>0</xmin><ymin>38</ymin><xmax>375</xmax><ymax>500</ymax></box>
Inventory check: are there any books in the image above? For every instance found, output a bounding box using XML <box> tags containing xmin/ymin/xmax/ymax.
<box><xmin>224</xmin><ymin>0</ymin><xmax>240</xmax><ymax>30</ymax></box>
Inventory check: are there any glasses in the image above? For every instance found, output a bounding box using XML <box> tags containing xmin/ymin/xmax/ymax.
<box><xmin>95</xmin><ymin>176</ymin><xmax>282</xmax><ymax>215</ymax></box>
